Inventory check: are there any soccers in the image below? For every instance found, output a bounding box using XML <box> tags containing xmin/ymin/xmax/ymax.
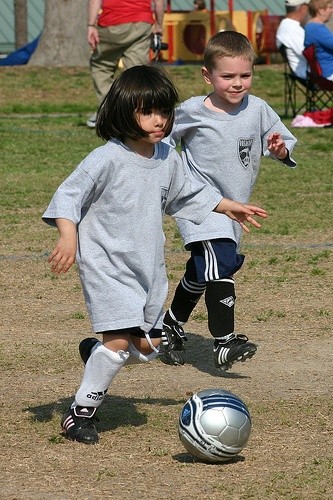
<box><xmin>177</xmin><ymin>388</ymin><xmax>251</xmax><ymax>465</ymax></box>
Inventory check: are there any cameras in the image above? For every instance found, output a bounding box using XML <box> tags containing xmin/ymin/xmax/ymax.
<box><xmin>151</xmin><ymin>33</ymin><xmax>168</xmax><ymax>51</ymax></box>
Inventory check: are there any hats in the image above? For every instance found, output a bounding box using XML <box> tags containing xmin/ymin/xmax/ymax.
<box><xmin>285</xmin><ymin>0</ymin><xmax>310</xmax><ymax>6</ymax></box>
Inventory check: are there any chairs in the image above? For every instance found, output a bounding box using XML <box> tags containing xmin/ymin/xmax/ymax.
<box><xmin>278</xmin><ymin>44</ymin><xmax>318</xmax><ymax>117</ymax></box>
<box><xmin>303</xmin><ymin>45</ymin><xmax>333</xmax><ymax>113</ymax></box>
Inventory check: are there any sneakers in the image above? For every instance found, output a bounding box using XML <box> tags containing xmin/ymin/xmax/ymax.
<box><xmin>78</xmin><ymin>337</ymin><xmax>102</xmax><ymax>366</ymax></box>
<box><xmin>212</xmin><ymin>333</ymin><xmax>258</xmax><ymax>373</ymax></box>
<box><xmin>156</xmin><ymin>307</ymin><xmax>188</xmax><ymax>366</ymax></box>
<box><xmin>60</xmin><ymin>405</ymin><xmax>100</xmax><ymax>444</ymax></box>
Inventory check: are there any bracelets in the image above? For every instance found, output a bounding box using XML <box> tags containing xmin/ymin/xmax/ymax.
<box><xmin>88</xmin><ymin>24</ymin><xmax>95</xmax><ymax>26</ymax></box>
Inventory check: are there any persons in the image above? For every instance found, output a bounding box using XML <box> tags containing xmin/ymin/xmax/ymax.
<box><xmin>191</xmin><ymin>0</ymin><xmax>208</xmax><ymax>13</ymax></box>
<box><xmin>276</xmin><ymin>0</ymin><xmax>311</xmax><ymax>87</ymax></box>
<box><xmin>42</xmin><ymin>65</ymin><xmax>267</xmax><ymax>444</ymax></box>
<box><xmin>160</xmin><ymin>31</ymin><xmax>297</xmax><ymax>372</ymax></box>
<box><xmin>304</xmin><ymin>0</ymin><xmax>333</xmax><ymax>82</ymax></box>
<box><xmin>86</xmin><ymin>0</ymin><xmax>164</xmax><ymax>128</ymax></box>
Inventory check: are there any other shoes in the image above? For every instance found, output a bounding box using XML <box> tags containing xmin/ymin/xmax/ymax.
<box><xmin>85</xmin><ymin>111</ymin><xmax>98</xmax><ymax>128</ymax></box>
